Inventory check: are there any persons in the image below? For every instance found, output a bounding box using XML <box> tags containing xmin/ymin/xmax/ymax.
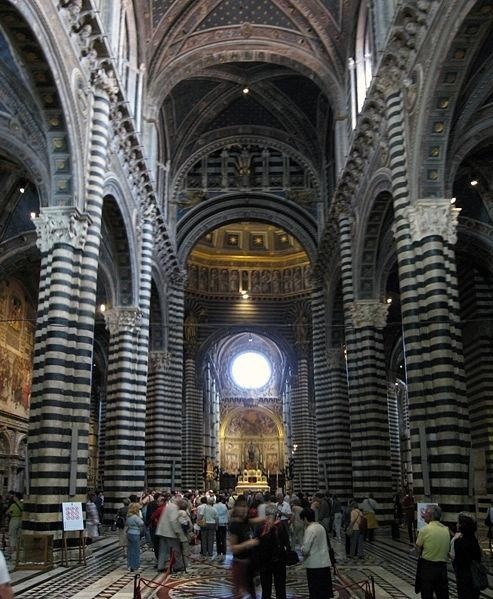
<box><xmin>117</xmin><ymin>487</ymin><xmax>228</xmax><ymax>571</ymax></box>
<box><xmin>227</xmin><ymin>488</ymin><xmax>343</xmax><ymax>548</ymax></box>
<box><xmin>296</xmin><ymin>508</ymin><xmax>334</xmax><ymax>599</ymax></box>
<box><xmin>393</xmin><ymin>490</ymin><xmax>417</xmax><ymax>543</ymax></box>
<box><xmin>253</xmin><ymin>505</ymin><xmax>290</xmax><ymax>599</ymax></box>
<box><xmin>0</xmin><ymin>490</ymin><xmax>23</xmax><ymax>553</ymax></box>
<box><xmin>85</xmin><ymin>491</ymin><xmax>104</xmax><ymax>542</ymax></box>
<box><xmin>485</xmin><ymin>507</ymin><xmax>492</xmax><ymax>549</ymax></box>
<box><xmin>343</xmin><ymin>494</ymin><xmax>376</xmax><ymax>559</ymax></box>
<box><xmin>449</xmin><ymin>512</ymin><xmax>482</xmax><ymax>599</ymax></box>
<box><xmin>228</xmin><ymin>495</ymin><xmax>268</xmax><ymax>599</ymax></box>
<box><xmin>415</xmin><ymin>505</ymin><xmax>450</xmax><ymax>599</ymax></box>
<box><xmin>1</xmin><ymin>553</ymin><xmax>13</xmax><ymax>599</ymax></box>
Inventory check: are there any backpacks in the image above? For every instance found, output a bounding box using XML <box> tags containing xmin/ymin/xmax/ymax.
<box><xmin>357</xmin><ymin>512</ymin><xmax>367</xmax><ymax>533</ymax></box>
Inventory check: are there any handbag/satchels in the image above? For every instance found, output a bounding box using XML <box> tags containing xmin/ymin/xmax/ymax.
<box><xmin>329</xmin><ymin>547</ymin><xmax>336</xmax><ymax>566</ymax></box>
<box><xmin>345</xmin><ymin>526</ymin><xmax>353</xmax><ymax>536</ymax></box>
<box><xmin>466</xmin><ymin>561</ymin><xmax>489</xmax><ymax>592</ymax></box>
<box><xmin>414</xmin><ymin>556</ymin><xmax>422</xmax><ymax>594</ymax></box>
<box><xmin>118</xmin><ymin>530</ymin><xmax>127</xmax><ymax>548</ymax></box>
<box><xmin>283</xmin><ymin>550</ymin><xmax>298</xmax><ymax>566</ymax></box>
<box><xmin>195</xmin><ymin>517</ymin><xmax>206</xmax><ymax>528</ymax></box>
